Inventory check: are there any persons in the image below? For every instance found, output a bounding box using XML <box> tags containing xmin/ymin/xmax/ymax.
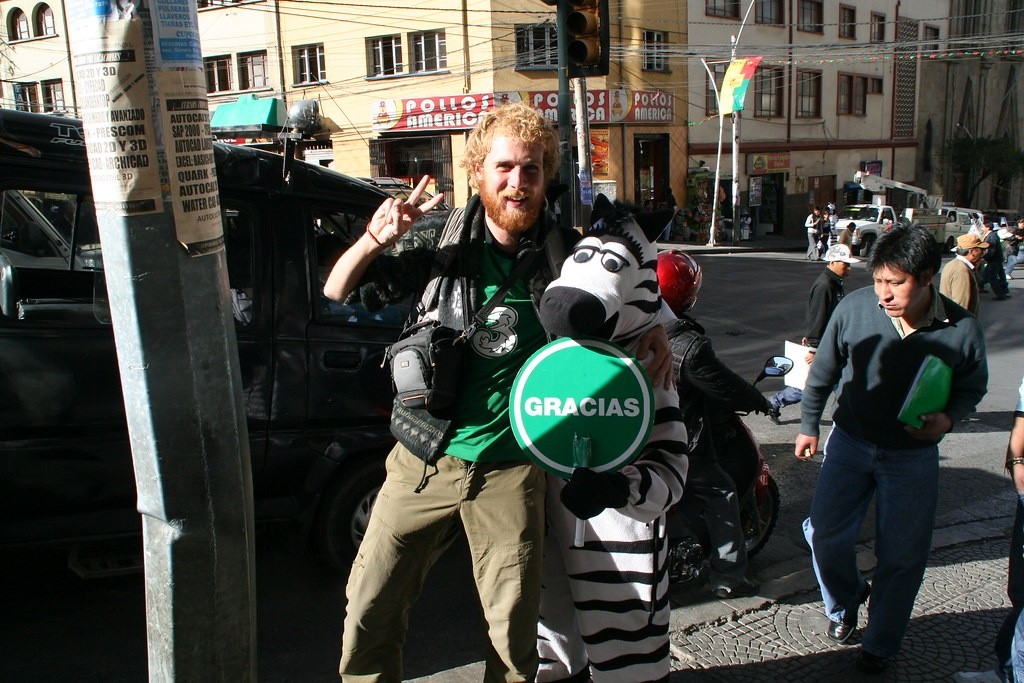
<box><xmin>322</xmin><ymin>105</ymin><xmax>675</xmax><ymax>683</ymax></box>
<box><xmin>951</xmin><ymin>359</ymin><xmax>1024</xmax><ymax>683</ymax></box>
<box><xmin>794</xmin><ymin>226</ymin><xmax>989</xmax><ymax>683</ymax></box>
<box><xmin>803</xmin><ymin>202</ymin><xmax>1024</xmax><ymax>301</ymax></box>
<box><xmin>938</xmin><ymin>232</ymin><xmax>990</xmax><ymax>317</ymax></box>
<box><xmin>767</xmin><ymin>244</ymin><xmax>862</xmax><ymax>426</ymax></box>
<box><xmin>656</xmin><ymin>247</ymin><xmax>776</xmax><ymax>598</ymax></box>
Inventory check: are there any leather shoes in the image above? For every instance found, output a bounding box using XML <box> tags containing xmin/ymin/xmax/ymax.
<box><xmin>856</xmin><ymin>650</ymin><xmax>890</xmax><ymax>674</ymax></box>
<box><xmin>827</xmin><ymin>580</ymin><xmax>872</xmax><ymax>642</ymax></box>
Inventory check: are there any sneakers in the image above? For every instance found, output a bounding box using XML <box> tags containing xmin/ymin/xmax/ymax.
<box><xmin>952</xmin><ymin>669</ymin><xmax>1011</xmax><ymax>683</ymax></box>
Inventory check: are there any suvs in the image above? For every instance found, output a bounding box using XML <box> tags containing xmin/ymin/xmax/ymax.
<box><xmin>0</xmin><ymin>105</ymin><xmax>446</xmax><ymax>582</ymax></box>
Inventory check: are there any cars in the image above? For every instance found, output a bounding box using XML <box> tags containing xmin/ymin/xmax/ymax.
<box><xmin>358</xmin><ymin>177</ymin><xmax>453</xmax><ymax>229</ymax></box>
<box><xmin>833</xmin><ymin>171</ymin><xmax>1013</xmax><ymax>258</ymax></box>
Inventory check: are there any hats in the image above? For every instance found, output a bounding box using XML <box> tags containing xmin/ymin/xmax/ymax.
<box><xmin>956</xmin><ymin>231</ymin><xmax>990</xmax><ymax>251</ymax></box>
<box><xmin>825</xmin><ymin>243</ymin><xmax>862</xmax><ymax>265</ymax></box>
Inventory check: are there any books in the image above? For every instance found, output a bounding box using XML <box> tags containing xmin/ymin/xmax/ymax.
<box><xmin>896</xmin><ymin>354</ymin><xmax>952</xmax><ymax>440</ymax></box>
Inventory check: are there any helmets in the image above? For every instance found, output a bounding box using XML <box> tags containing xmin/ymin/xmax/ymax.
<box><xmin>656</xmin><ymin>248</ymin><xmax>703</xmax><ymax>315</ymax></box>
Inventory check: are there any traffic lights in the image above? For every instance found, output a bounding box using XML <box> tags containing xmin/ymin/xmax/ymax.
<box><xmin>567</xmin><ymin>0</ymin><xmax>601</xmax><ymax>69</ymax></box>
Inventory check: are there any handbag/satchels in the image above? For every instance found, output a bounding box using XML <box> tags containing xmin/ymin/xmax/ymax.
<box><xmin>378</xmin><ymin>319</ymin><xmax>468</xmax><ymax>419</ymax></box>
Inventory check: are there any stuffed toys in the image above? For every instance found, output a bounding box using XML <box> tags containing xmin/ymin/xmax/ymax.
<box><xmin>501</xmin><ymin>195</ymin><xmax>693</xmax><ymax>683</ymax></box>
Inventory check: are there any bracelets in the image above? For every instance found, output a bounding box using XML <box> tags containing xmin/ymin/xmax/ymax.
<box><xmin>367</xmin><ymin>222</ymin><xmax>390</xmax><ymax>248</ymax></box>
<box><xmin>1005</xmin><ymin>457</ymin><xmax>1024</xmax><ymax>474</ymax></box>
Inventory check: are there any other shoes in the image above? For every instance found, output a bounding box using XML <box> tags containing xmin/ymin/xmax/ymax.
<box><xmin>979</xmin><ymin>286</ymin><xmax>988</xmax><ymax>293</ymax></box>
<box><xmin>715</xmin><ymin>574</ymin><xmax>761</xmax><ymax>600</ymax></box>
<box><xmin>992</xmin><ymin>293</ymin><xmax>1010</xmax><ymax>300</ymax></box>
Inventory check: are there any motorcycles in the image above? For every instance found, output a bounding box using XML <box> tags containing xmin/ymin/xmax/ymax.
<box><xmin>667</xmin><ymin>355</ymin><xmax>796</xmax><ymax>589</ymax></box>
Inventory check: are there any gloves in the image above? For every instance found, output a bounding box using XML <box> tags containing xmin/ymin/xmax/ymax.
<box><xmin>769</xmin><ymin>404</ymin><xmax>781</xmax><ymax>417</ymax></box>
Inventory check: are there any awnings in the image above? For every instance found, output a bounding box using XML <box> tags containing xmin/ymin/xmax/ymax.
<box><xmin>209</xmin><ymin>94</ymin><xmax>290</xmax><ymax>136</ymax></box>
